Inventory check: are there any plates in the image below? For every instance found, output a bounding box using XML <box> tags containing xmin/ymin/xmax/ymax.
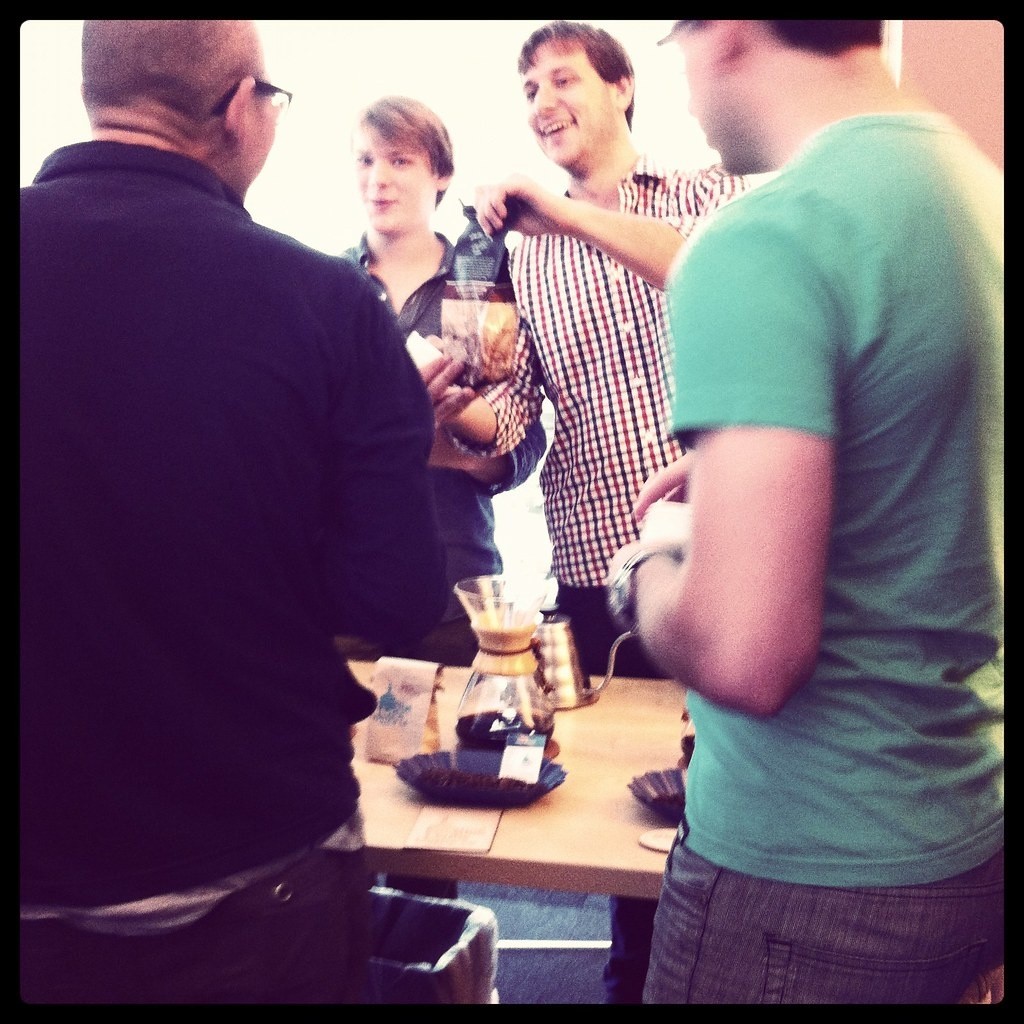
<box><xmin>629</xmin><ymin>768</ymin><xmax>685</xmax><ymax>819</ymax></box>
<box><xmin>393</xmin><ymin>751</ymin><xmax>567</xmax><ymax>806</ymax></box>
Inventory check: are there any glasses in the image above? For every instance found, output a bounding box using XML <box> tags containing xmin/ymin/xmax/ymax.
<box><xmin>210</xmin><ymin>79</ymin><xmax>293</xmax><ymax>116</ymax></box>
<box><xmin>657</xmin><ymin>20</ymin><xmax>697</xmax><ymax>73</ymax></box>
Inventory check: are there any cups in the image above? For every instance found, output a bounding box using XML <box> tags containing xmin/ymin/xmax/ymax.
<box><xmin>364</xmin><ymin>658</ymin><xmax>438</xmax><ymax>765</ymax></box>
<box><xmin>640</xmin><ymin>501</ymin><xmax>693</xmax><ymax>542</ymax></box>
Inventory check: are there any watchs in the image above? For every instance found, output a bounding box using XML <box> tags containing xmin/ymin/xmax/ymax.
<box><xmin>603</xmin><ymin>536</ymin><xmax>685</xmax><ymax>637</ymax></box>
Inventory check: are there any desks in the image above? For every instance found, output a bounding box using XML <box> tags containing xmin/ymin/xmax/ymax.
<box><xmin>344</xmin><ymin>658</ymin><xmax>696</xmax><ymax>898</ymax></box>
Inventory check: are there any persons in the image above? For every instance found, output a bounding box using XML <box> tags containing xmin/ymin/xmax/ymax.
<box><xmin>21</xmin><ymin>20</ymin><xmax>1004</xmax><ymax>1004</ymax></box>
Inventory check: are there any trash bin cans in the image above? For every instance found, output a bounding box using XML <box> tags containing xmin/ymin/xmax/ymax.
<box><xmin>369</xmin><ymin>886</ymin><xmax>497</xmax><ymax>1004</ymax></box>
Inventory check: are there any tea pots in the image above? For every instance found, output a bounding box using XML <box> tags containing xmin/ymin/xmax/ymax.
<box><xmin>535</xmin><ymin>602</ymin><xmax>633</xmax><ymax>709</ymax></box>
